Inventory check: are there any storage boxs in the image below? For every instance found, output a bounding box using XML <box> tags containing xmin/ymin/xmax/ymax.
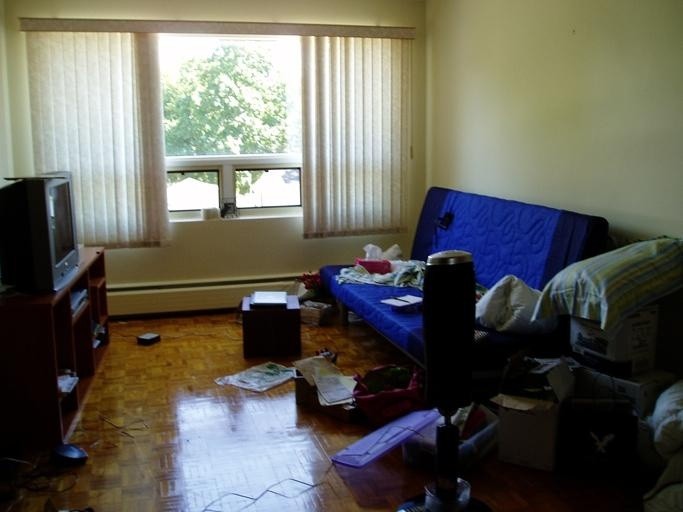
<box><xmin>491</xmin><ymin>302</ymin><xmax>674</xmax><ymax>470</ymax></box>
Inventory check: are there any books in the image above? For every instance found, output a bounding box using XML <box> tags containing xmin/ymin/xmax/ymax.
<box><xmin>248</xmin><ymin>290</ymin><xmax>288</xmax><ymax>309</ymax></box>
<box><xmin>379</xmin><ymin>293</ymin><xmax>423</xmax><ymax>306</ymax></box>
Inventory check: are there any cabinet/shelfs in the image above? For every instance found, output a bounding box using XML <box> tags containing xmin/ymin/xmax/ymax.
<box><xmin>0</xmin><ymin>245</ymin><xmax>110</xmax><ymax>450</ymax></box>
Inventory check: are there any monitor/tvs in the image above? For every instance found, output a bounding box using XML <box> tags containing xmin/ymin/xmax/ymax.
<box><xmin>0</xmin><ymin>177</ymin><xmax>80</xmax><ymax>294</ymax></box>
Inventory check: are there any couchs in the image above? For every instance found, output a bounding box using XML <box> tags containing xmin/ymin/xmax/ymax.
<box><xmin>318</xmin><ymin>184</ymin><xmax>609</xmax><ymax>410</ymax></box>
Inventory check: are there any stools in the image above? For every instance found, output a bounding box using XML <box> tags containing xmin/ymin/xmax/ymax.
<box><xmin>240</xmin><ymin>295</ymin><xmax>301</xmax><ymax>359</ymax></box>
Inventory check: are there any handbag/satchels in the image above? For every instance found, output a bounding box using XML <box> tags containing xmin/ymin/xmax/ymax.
<box><xmin>353</xmin><ymin>363</ymin><xmax>423</xmax><ymax>428</ymax></box>
<box><xmin>555</xmin><ymin>376</ymin><xmax>637</xmax><ymax>499</ymax></box>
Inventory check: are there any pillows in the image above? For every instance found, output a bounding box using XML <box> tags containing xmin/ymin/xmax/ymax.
<box><xmin>530</xmin><ymin>235</ymin><xmax>683</xmax><ymax>331</ymax></box>
